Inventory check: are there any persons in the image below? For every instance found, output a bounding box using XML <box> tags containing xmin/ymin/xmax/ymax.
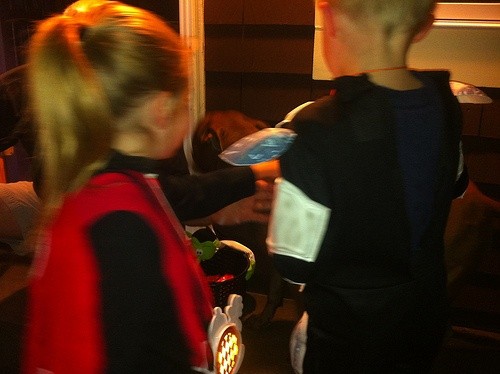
<box><xmin>267</xmin><ymin>0</ymin><xmax>470</xmax><ymax>374</ymax></box>
<box><xmin>32</xmin><ymin>135</ymin><xmax>280</xmax><ymax>229</ymax></box>
<box><xmin>21</xmin><ymin>0</ymin><xmax>218</xmax><ymax>374</ymax></box>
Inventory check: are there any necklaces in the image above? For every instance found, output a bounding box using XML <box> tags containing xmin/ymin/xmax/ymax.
<box><xmin>343</xmin><ymin>66</ymin><xmax>408</xmax><ymax>75</ymax></box>
